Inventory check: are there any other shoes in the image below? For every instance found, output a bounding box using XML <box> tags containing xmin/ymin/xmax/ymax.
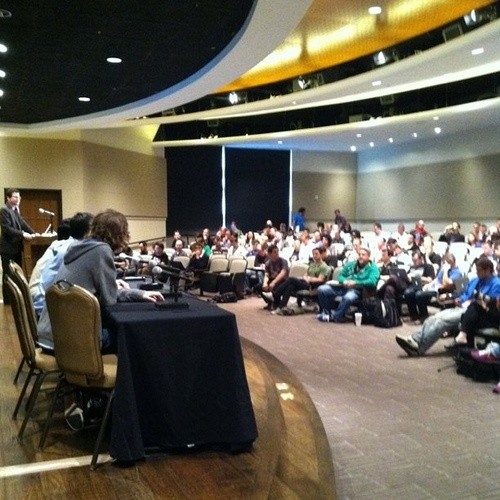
<box><xmin>403</xmin><ymin>316</ymin><xmax>411</xmax><ymax>323</ymax></box>
<box><xmin>444</xmin><ymin>337</ymin><xmax>468</xmax><ymax>350</ymax></box>
<box><xmin>492</xmin><ymin>382</ymin><xmax>500</xmax><ymax>393</ymax></box>
<box><xmin>414</xmin><ymin>316</ymin><xmax>424</xmax><ymax>324</ymax></box>
<box><xmin>264</xmin><ymin>302</ymin><xmax>276</xmax><ymax>310</ymax></box>
<box><xmin>477</xmin><ymin>342</ymin><xmax>486</xmax><ymax>350</ymax></box>
<box><xmin>87</xmin><ymin>388</ymin><xmax>103</xmax><ymax>422</ymax></box>
<box><xmin>64</xmin><ymin>390</ymin><xmax>84</xmax><ymax>431</ymax></box>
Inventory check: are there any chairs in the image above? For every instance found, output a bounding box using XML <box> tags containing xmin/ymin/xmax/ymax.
<box><xmin>44</xmin><ymin>282</ymin><xmax>119</xmax><ymax>471</ymax></box>
<box><xmin>288</xmin><ymin>264</ymin><xmax>310</xmax><ymax>278</ymax></box>
<box><xmin>220</xmin><ymin>260</ymin><xmax>247</xmax><ymax>293</ymax></box>
<box><xmin>466</xmin><ymin>328</ymin><xmax>499</xmax><ymax>349</ymax></box>
<box><xmin>4</xmin><ymin>276</ymin><xmax>56</xmax><ymax>441</ymax></box>
<box><xmin>298</xmin><ymin>267</ymin><xmax>333</xmax><ymax>308</ymax></box>
<box><xmin>173</xmin><ymin>256</ymin><xmax>189</xmax><ymax>269</ymax></box>
<box><xmin>246</xmin><ymin>256</ymin><xmax>257</xmax><ymax>269</ymax></box>
<box><xmin>4</xmin><ymin>260</ymin><xmax>37</xmax><ymax>383</ymax></box>
<box><xmin>208</xmin><ymin>254</ymin><xmax>226</xmax><ymax>265</ymax></box>
<box><xmin>198</xmin><ymin>260</ymin><xmax>228</xmax><ymax>295</ymax></box>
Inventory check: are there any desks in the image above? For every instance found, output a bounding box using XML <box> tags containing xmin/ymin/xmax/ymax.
<box><xmin>100</xmin><ymin>276</ymin><xmax>259</xmax><ymax>464</ymax></box>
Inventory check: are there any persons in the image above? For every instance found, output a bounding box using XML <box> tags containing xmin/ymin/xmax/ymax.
<box><xmin>29</xmin><ymin>218</ymin><xmax>72</xmax><ymax>298</ymax></box>
<box><xmin>33</xmin><ymin>212</ymin><xmax>127</xmax><ymax>319</ymax></box>
<box><xmin>36</xmin><ymin>209</ymin><xmax>165</xmax><ymax>432</ymax></box>
<box><xmin>117</xmin><ymin>205</ymin><xmax>500</xmax><ymax>394</ymax></box>
<box><xmin>0</xmin><ymin>187</ymin><xmax>39</xmax><ymax>304</ymax></box>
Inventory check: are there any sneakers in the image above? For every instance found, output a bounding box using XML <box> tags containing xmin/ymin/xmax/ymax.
<box><xmin>270</xmin><ymin>307</ymin><xmax>283</xmax><ymax>315</ymax></box>
<box><xmin>318</xmin><ymin>312</ymin><xmax>333</xmax><ymax>322</ymax></box>
<box><xmin>395</xmin><ymin>334</ymin><xmax>419</xmax><ymax>358</ymax></box>
<box><xmin>262</xmin><ymin>291</ymin><xmax>274</xmax><ymax>302</ymax></box>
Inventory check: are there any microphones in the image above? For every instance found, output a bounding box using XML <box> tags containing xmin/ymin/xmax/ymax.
<box><xmin>158</xmin><ymin>261</ymin><xmax>180</xmax><ymax>274</ymax></box>
<box><xmin>152</xmin><ymin>266</ymin><xmax>189</xmax><ymax>280</ymax></box>
<box><xmin>131</xmin><ymin>254</ymin><xmax>154</xmax><ymax>264</ymax></box>
<box><xmin>118</xmin><ymin>252</ymin><xmax>132</xmax><ymax>261</ymax></box>
<box><xmin>149</xmin><ymin>260</ymin><xmax>160</xmax><ymax>268</ymax></box>
<box><xmin>38</xmin><ymin>207</ymin><xmax>55</xmax><ymax>217</ymax></box>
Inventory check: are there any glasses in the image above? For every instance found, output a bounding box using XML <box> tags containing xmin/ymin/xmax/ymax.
<box><xmin>11</xmin><ymin>195</ymin><xmax>21</xmax><ymax>199</ymax></box>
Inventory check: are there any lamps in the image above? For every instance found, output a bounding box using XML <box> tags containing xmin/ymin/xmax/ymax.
<box><xmin>228</xmin><ymin>91</ymin><xmax>248</xmax><ymax>104</ymax></box>
<box><xmin>298</xmin><ymin>77</ymin><xmax>313</xmax><ymax>89</ymax></box>
<box><xmin>464</xmin><ymin>10</ymin><xmax>494</xmax><ymax>25</ymax></box>
<box><xmin>373</xmin><ymin>49</ymin><xmax>398</xmax><ymax>66</ymax></box>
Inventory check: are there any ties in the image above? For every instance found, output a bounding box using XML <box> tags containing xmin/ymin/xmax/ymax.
<box><xmin>14</xmin><ymin>207</ymin><xmax>21</xmax><ymax>228</ymax></box>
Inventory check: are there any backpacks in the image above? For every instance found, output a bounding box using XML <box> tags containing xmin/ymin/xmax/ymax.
<box><xmin>454</xmin><ymin>344</ymin><xmax>500</xmax><ymax>381</ymax></box>
<box><xmin>360</xmin><ymin>297</ymin><xmax>402</xmax><ymax>327</ymax></box>
